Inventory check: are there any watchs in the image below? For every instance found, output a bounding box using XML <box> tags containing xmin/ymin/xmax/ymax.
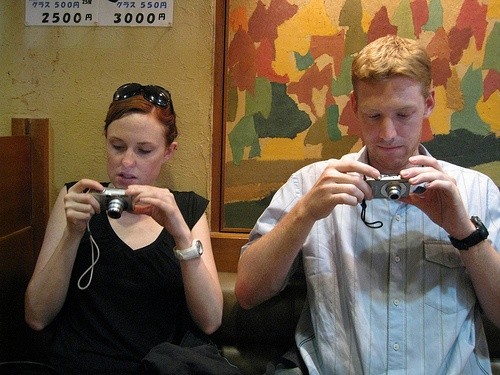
<box><xmin>448</xmin><ymin>216</ymin><xmax>489</xmax><ymax>250</ymax></box>
<box><xmin>174</xmin><ymin>239</ymin><xmax>204</xmax><ymax>261</ymax></box>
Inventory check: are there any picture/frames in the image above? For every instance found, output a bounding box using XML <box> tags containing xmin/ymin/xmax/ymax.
<box><xmin>210</xmin><ymin>0</ymin><xmax>500</xmax><ymax>239</ymax></box>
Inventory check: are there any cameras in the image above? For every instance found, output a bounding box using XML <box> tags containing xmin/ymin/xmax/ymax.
<box><xmin>365</xmin><ymin>173</ymin><xmax>411</xmax><ymax>201</ymax></box>
<box><xmin>87</xmin><ymin>187</ymin><xmax>133</xmax><ymax>219</ymax></box>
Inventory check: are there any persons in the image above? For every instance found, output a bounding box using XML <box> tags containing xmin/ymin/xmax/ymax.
<box><xmin>24</xmin><ymin>83</ymin><xmax>224</xmax><ymax>375</ymax></box>
<box><xmin>235</xmin><ymin>33</ymin><xmax>500</xmax><ymax>375</ymax></box>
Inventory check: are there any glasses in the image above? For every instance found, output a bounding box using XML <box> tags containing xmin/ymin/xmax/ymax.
<box><xmin>113</xmin><ymin>82</ymin><xmax>174</xmax><ymax>114</ymax></box>
